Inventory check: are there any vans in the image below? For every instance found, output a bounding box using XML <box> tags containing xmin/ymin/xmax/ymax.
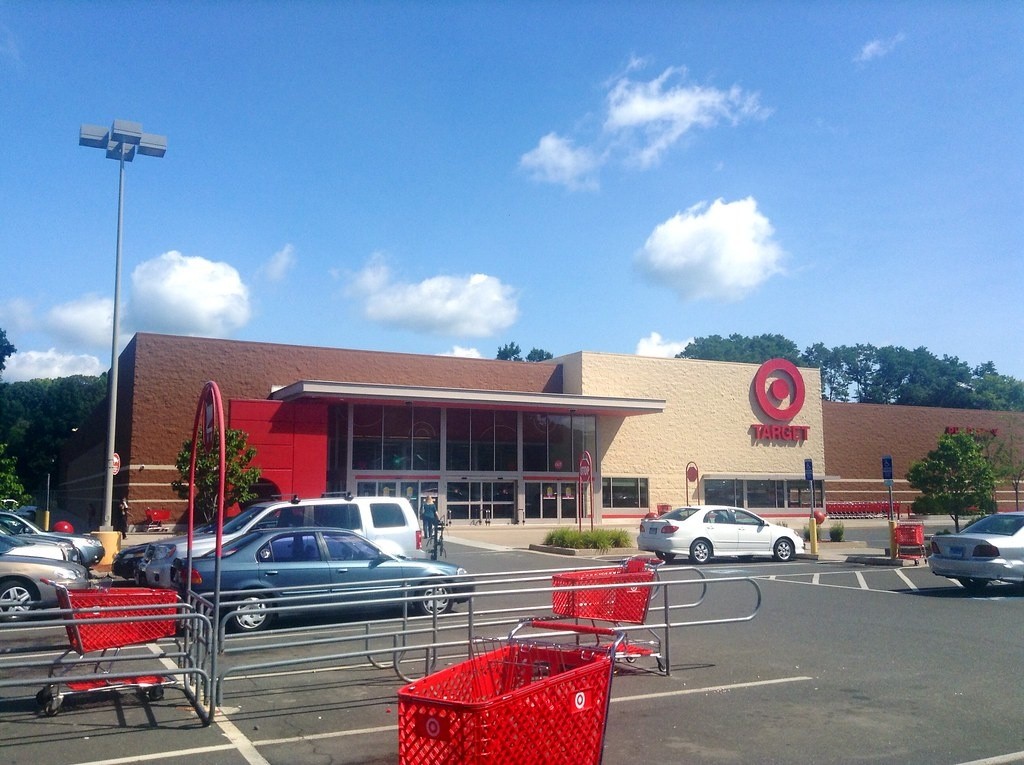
<box><xmin>111</xmin><ymin>492</ymin><xmax>427</xmax><ymax>589</ymax></box>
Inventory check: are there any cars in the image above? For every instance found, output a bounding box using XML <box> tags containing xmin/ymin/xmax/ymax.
<box><xmin>0</xmin><ymin>499</ymin><xmax>105</xmax><ymax>623</ymax></box>
<box><xmin>928</xmin><ymin>511</ymin><xmax>1024</xmax><ymax>588</ymax></box>
<box><xmin>637</xmin><ymin>505</ymin><xmax>806</xmax><ymax>565</ymax></box>
<box><xmin>173</xmin><ymin>527</ymin><xmax>474</xmax><ymax>632</ymax></box>
<box><xmin>421</xmin><ymin>487</ymin><xmax>463</xmax><ymax>501</ymax></box>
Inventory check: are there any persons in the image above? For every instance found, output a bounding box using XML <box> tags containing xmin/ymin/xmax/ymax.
<box><xmin>118</xmin><ymin>496</ymin><xmax>134</xmax><ymax>539</ymax></box>
<box><xmin>422</xmin><ymin>494</ymin><xmax>441</xmax><ymax>538</ymax></box>
<box><xmin>87</xmin><ymin>503</ymin><xmax>97</xmax><ymax>524</ymax></box>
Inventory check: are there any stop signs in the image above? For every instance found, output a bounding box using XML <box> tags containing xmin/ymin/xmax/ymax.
<box><xmin>579</xmin><ymin>458</ymin><xmax>590</xmax><ymax>482</ymax></box>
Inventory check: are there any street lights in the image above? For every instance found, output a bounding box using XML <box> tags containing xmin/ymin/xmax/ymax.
<box><xmin>79</xmin><ymin>120</ymin><xmax>167</xmax><ymax>533</ymax></box>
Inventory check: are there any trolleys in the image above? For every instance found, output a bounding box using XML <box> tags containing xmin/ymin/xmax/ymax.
<box><xmin>895</xmin><ymin>519</ymin><xmax>927</xmax><ymax>566</ymax></box>
<box><xmin>825</xmin><ymin>500</ymin><xmax>901</xmax><ymax>519</ymax></box>
<box><xmin>552</xmin><ymin>558</ymin><xmax>668</xmax><ymax>672</ymax></box>
<box><xmin>145</xmin><ymin>509</ymin><xmax>170</xmax><ymax>531</ymax></box>
<box><xmin>37</xmin><ymin>577</ymin><xmax>177</xmax><ymax>717</ymax></box>
<box><xmin>397</xmin><ymin>621</ymin><xmax>625</xmax><ymax>765</ymax></box>
